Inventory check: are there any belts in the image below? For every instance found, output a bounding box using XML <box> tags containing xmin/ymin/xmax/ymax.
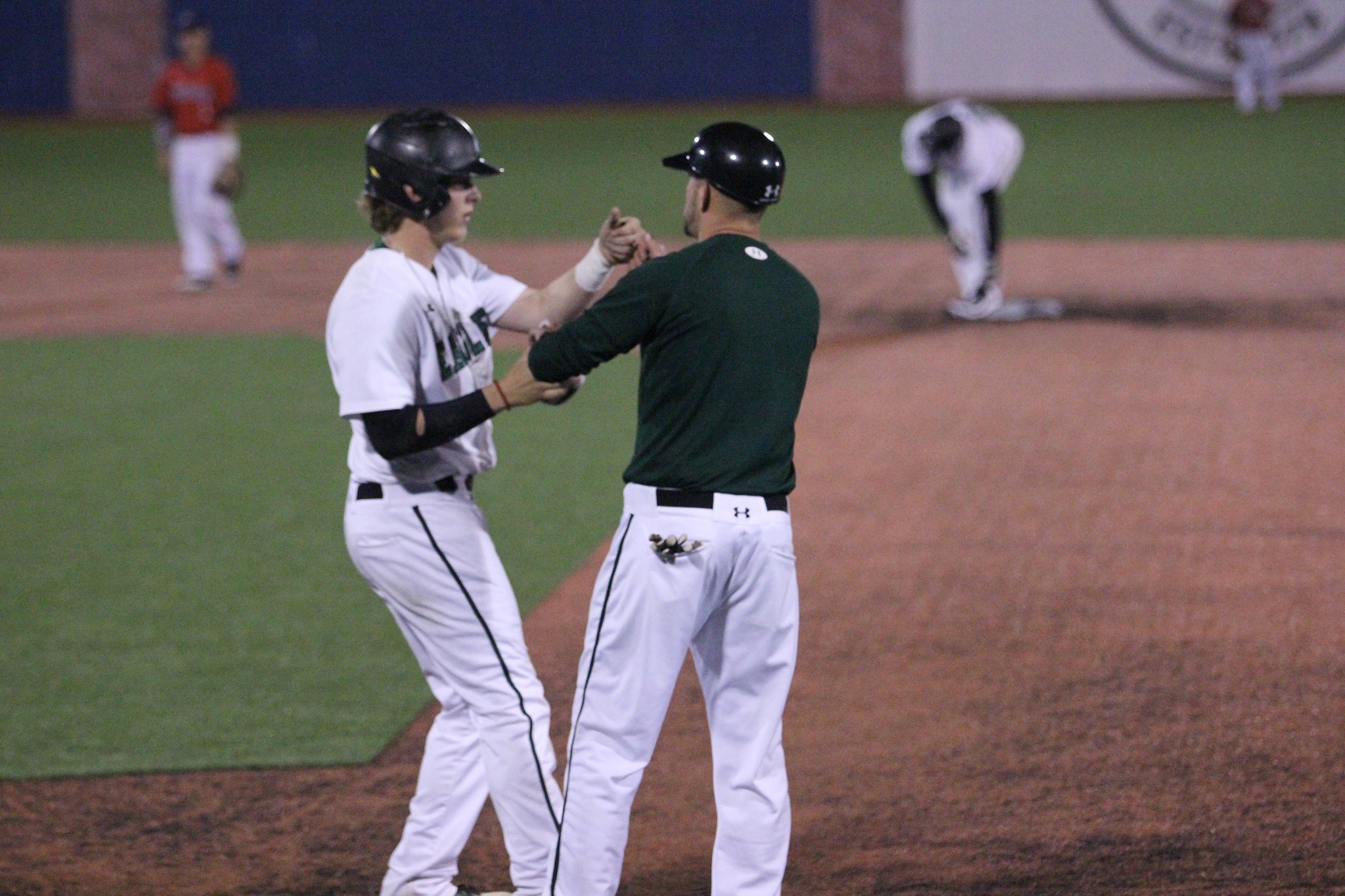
<box><xmin>357</xmin><ymin>474</ymin><xmax>474</xmax><ymax>501</ymax></box>
<box><xmin>654</xmin><ymin>487</ymin><xmax>788</xmax><ymax>512</ymax></box>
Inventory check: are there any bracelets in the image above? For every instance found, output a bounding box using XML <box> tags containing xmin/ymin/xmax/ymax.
<box><xmin>574</xmin><ymin>237</ymin><xmax>615</xmax><ymax>293</ymax></box>
<box><xmin>492</xmin><ymin>378</ymin><xmax>512</xmax><ymax>412</ymax></box>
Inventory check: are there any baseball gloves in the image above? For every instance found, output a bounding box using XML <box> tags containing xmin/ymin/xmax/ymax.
<box><xmin>210</xmin><ymin>161</ymin><xmax>244</xmax><ymax>200</ymax></box>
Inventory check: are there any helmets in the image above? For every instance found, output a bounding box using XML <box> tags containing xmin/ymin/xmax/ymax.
<box><xmin>661</xmin><ymin>120</ymin><xmax>786</xmax><ymax>209</ymax></box>
<box><xmin>921</xmin><ymin>117</ymin><xmax>961</xmax><ymax>152</ymax></box>
<box><xmin>362</xmin><ymin>109</ymin><xmax>504</xmax><ymax>221</ymax></box>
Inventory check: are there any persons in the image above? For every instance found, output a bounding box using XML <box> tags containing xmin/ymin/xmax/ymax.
<box><xmin>1225</xmin><ymin>0</ymin><xmax>1283</xmax><ymax>114</ymax></box>
<box><xmin>317</xmin><ymin>106</ymin><xmax>647</xmax><ymax>896</ymax></box>
<box><xmin>899</xmin><ymin>97</ymin><xmax>1025</xmax><ymax>323</ymax></box>
<box><xmin>528</xmin><ymin>121</ymin><xmax>821</xmax><ymax>896</ymax></box>
<box><xmin>151</xmin><ymin>10</ymin><xmax>250</xmax><ymax>293</ymax></box>
